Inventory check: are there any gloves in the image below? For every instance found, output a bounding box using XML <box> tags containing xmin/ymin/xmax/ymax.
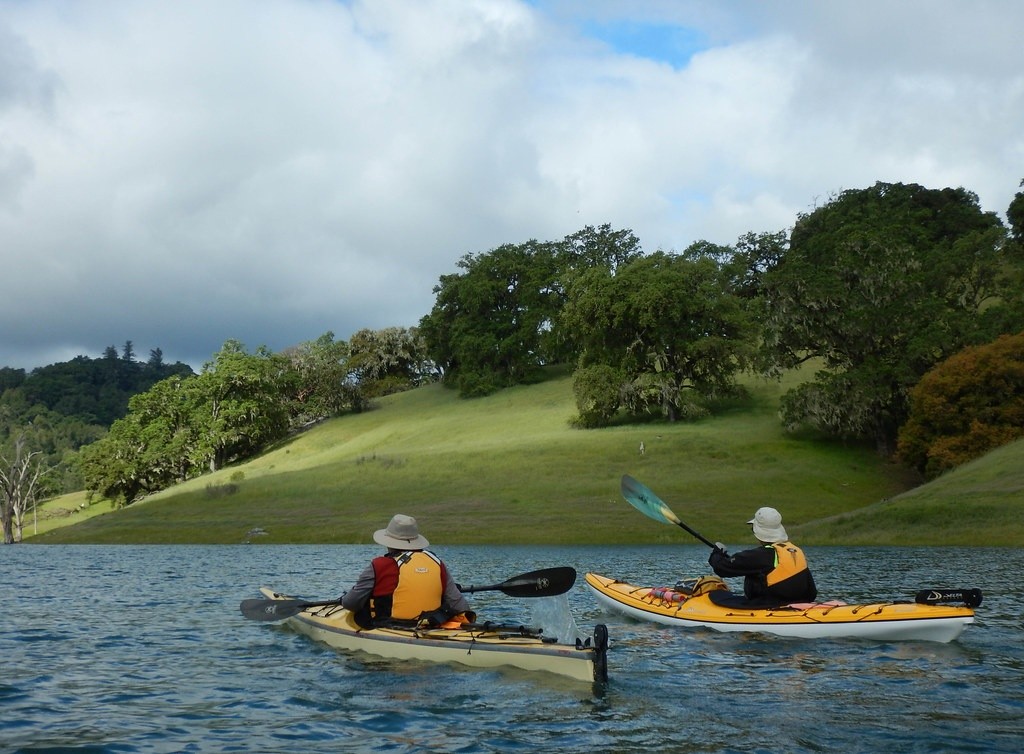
<box><xmin>715</xmin><ymin>542</ymin><xmax>727</xmax><ymax>553</ymax></box>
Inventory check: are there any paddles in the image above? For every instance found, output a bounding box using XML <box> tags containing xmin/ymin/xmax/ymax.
<box><xmin>619</xmin><ymin>472</ymin><xmax>719</xmax><ymax>548</ymax></box>
<box><xmin>241</xmin><ymin>566</ymin><xmax>576</xmax><ymax>622</ymax></box>
<box><xmin>439</xmin><ymin>617</ymin><xmax>544</xmax><ymax>634</ymax></box>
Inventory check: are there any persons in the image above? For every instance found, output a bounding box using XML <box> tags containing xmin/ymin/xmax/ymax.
<box><xmin>708</xmin><ymin>506</ymin><xmax>819</xmax><ymax>608</ymax></box>
<box><xmin>341</xmin><ymin>513</ymin><xmax>471</xmax><ymax>622</ymax></box>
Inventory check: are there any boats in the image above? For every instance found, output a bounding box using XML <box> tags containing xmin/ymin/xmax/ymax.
<box><xmin>256</xmin><ymin>584</ymin><xmax>610</xmax><ymax>686</ymax></box>
<box><xmin>584</xmin><ymin>563</ymin><xmax>984</xmax><ymax>649</ymax></box>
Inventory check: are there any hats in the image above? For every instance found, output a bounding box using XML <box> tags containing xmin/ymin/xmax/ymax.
<box><xmin>373</xmin><ymin>514</ymin><xmax>429</xmax><ymax>550</ymax></box>
<box><xmin>746</xmin><ymin>507</ymin><xmax>788</xmax><ymax>543</ymax></box>
